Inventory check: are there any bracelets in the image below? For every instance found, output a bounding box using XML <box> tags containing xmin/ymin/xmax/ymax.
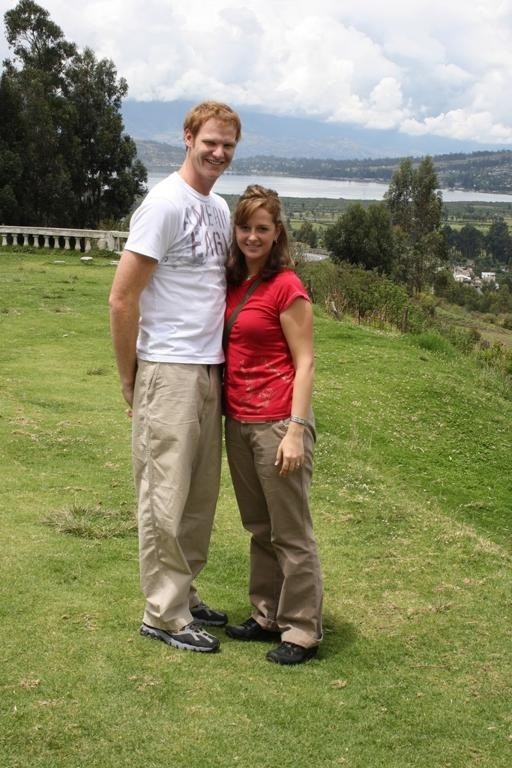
<box><xmin>290</xmin><ymin>415</ymin><xmax>308</xmax><ymax>425</ymax></box>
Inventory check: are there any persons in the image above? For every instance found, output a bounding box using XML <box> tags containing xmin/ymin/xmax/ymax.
<box><xmin>219</xmin><ymin>184</ymin><xmax>324</xmax><ymax>665</ymax></box>
<box><xmin>108</xmin><ymin>99</ymin><xmax>241</xmax><ymax>653</ymax></box>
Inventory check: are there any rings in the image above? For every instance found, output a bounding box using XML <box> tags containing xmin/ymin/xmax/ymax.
<box><xmin>296</xmin><ymin>463</ymin><xmax>301</xmax><ymax>467</ymax></box>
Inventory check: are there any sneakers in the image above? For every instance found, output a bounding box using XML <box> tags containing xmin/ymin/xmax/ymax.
<box><xmin>192</xmin><ymin>602</ymin><xmax>227</xmax><ymax>626</ymax></box>
<box><xmin>226</xmin><ymin>616</ymin><xmax>281</xmax><ymax>642</ymax></box>
<box><xmin>267</xmin><ymin>641</ymin><xmax>318</xmax><ymax>665</ymax></box>
<box><xmin>139</xmin><ymin>619</ymin><xmax>218</xmax><ymax>652</ymax></box>
